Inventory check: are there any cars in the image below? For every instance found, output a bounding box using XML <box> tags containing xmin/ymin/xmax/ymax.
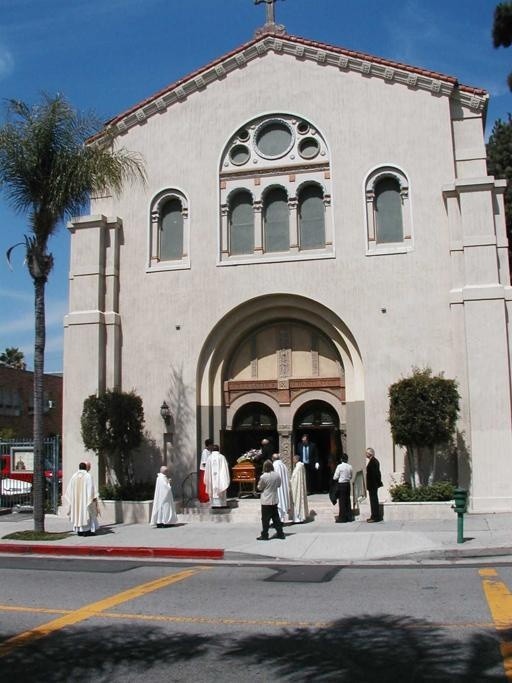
<box><xmin>0</xmin><ymin>475</ymin><xmax>32</xmax><ymax>503</ymax></box>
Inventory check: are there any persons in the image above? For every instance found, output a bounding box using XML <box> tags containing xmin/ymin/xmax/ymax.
<box><xmin>296</xmin><ymin>434</ymin><xmax>320</xmax><ymax>496</ymax></box>
<box><xmin>365</xmin><ymin>448</ymin><xmax>384</xmax><ymax>523</ymax></box>
<box><xmin>332</xmin><ymin>453</ymin><xmax>355</xmax><ymax>523</ymax></box>
<box><xmin>252</xmin><ymin>438</ymin><xmax>273</xmax><ymax>467</ymax></box>
<box><xmin>289</xmin><ymin>455</ymin><xmax>308</xmax><ymax>524</ymax></box>
<box><xmin>149</xmin><ymin>466</ymin><xmax>178</xmax><ymax>528</ymax></box>
<box><xmin>269</xmin><ymin>453</ymin><xmax>290</xmax><ymax>528</ymax></box>
<box><xmin>255</xmin><ymin>459</ymin><xmax>285</xmax><ymax>540</ymax></box>
<box><xmin>203</xmin><ymin>445</ymin><xmax>232</xmax><ymax>509</ymax></box>
<box><xmin>198</xmin><ymin>439</ymin><xmax>214</xmax><ymax>504</ymax></box>
<box><xmin>64</xmin><ymin>461</ymin><xmax>99</xmax><ymax>536</ymax></box>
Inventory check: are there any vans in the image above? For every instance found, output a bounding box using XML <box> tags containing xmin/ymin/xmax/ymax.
<box><xmin>0</xmin><ymin>453</ymin><xmax>61</xmax><ymax>491</ymax></box>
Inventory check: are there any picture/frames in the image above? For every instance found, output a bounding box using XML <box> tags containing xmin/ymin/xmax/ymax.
<box><xmin>10</xmin><ymin>447</ymin><xmax>34</xmax><ymax>475</ymax></box>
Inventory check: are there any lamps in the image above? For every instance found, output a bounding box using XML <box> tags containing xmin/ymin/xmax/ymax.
<box><xmin>160</xmin><ymin>400</ymin><xmax>170</xmax><ymax>420</ymax></box>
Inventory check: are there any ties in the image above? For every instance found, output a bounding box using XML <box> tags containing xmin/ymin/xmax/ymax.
<box><xmin>305</xmin><ymin>445</ymin><xmax>307</xmax><ymax>461</ymax></box>
